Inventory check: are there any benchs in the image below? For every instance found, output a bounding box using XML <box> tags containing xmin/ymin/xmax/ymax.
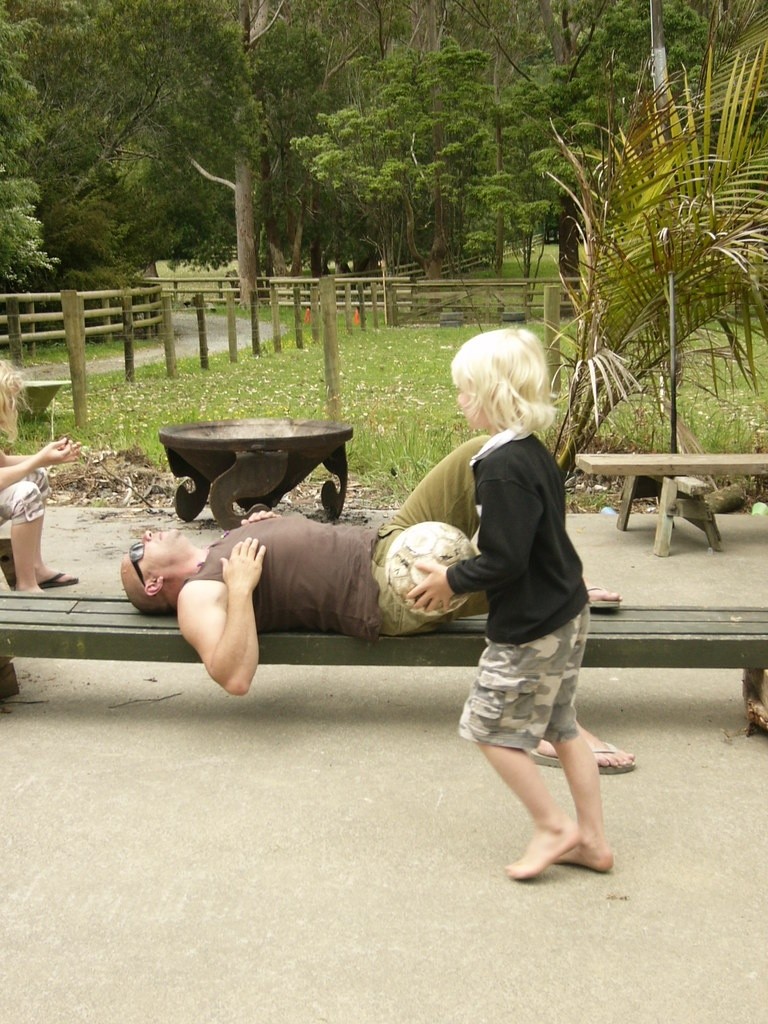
<box><xmin>574</xmin><ymin>452</ymin><xmax>768</xmax><ymax>558</ymax></box>
<box><xmin>0</xmin><ymin>590</ymin><xmax>768</xmax><ymax>736</ymax></box>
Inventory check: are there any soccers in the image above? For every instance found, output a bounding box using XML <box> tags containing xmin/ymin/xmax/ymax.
<box><xmin>384</xmin><ymin>520</ymin><xmax>478</xmax><ymax>617</ymax></box>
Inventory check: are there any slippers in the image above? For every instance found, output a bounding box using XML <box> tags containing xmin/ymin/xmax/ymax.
<box><xmin>585</xmin><ymin>587</ymin><xmax>624</xmax><ymax>610</ymax></box>
<box><xmin>531</xmin><ymin>742</ymin><xmax>637</xmax><ymax>774</ymax></box>
<box><xmin>33</xmin><ymin>571</ymin><xmax>79</xmax><ymax>589</ymax></box>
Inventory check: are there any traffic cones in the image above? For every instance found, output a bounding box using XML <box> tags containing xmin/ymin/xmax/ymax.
<box><xmin>303</xmin><ymin>307</ymin><xmax>313</xmax><ymax>325</ymax></box>
<box><xmin>353</xmin><ymin>308</ymin><xmax>361</xmax><ymax>325</ymax></box>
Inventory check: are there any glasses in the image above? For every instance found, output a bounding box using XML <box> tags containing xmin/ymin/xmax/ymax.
<box><xmin>129</xmin><ymin>543</ymin><xmax>146</xmax><ymax>588</ymax></box>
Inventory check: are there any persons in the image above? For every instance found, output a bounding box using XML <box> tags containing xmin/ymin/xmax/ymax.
<box><xmin>120</xmin><ymin>433</ymin><xmax>635</xmax><ymax>775</ymax></box>
<box><xmin>0</xmin><ymin>357</ymin><xmax>83</xmax><ymax>593</ymax></box>
<box><xmin>403</xmin><ymin>328</ymin><xmax>615</xmax><ymax>879</ymax></box>
